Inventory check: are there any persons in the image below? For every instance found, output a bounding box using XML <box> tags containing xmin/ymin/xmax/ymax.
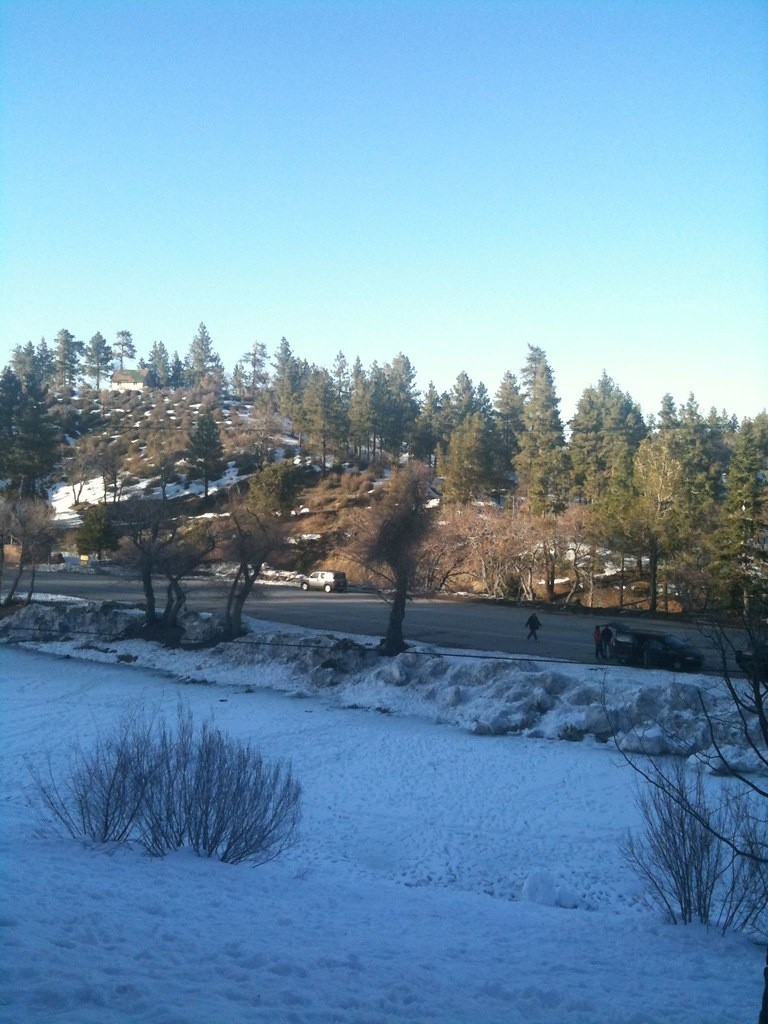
<box><xmin>601</xmin><ymin>626</ymin><xmax>613</xmax><ymax>658</ymax></box>
<box><xmin>593</xmin><ymin>625</ymin><xmax>604</xmax><ymax>659</ymax></box>
<box><xmin>525</xmin><ymin>612</ymin><xmax>542</xmax><ymax>642</ymax></box>
<box><xmin>642</xmin><ymin>636</ymin><xmax>657</xmax><ymax>668</ymax></box>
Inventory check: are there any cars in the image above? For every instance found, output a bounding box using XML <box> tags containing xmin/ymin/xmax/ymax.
<box><xmin>599</xmin><ymin>623</ymin><xmax>703</xmax><ymax>672</ymax></box>
<box><xmin>735</xmin><ymin>642</ymin><xmax>768</xmax><ymax>679</ymax></box>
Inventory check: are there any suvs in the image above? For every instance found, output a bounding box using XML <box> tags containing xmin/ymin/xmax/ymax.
<box><xmin>301</xmin><ymin>571</ymin><xmax>347</xmax><ymax>593</ymax></box>
